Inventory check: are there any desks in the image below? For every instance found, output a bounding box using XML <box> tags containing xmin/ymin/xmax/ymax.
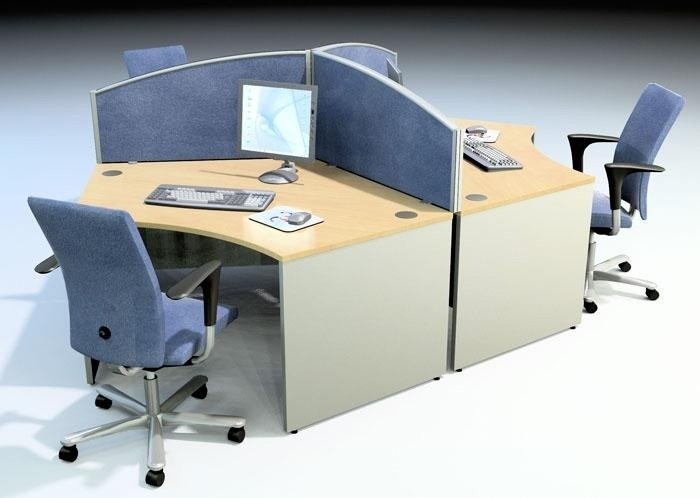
<box><xmin>79</xmin><ymin>118</ymin><xmax>594</xmax><ymax>433</ymax></box>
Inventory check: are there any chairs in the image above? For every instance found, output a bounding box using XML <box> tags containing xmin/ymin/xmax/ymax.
<box><xmin>125</xmin><ymin>45</ymin><xmax>190</xmax><ymax>79</ymax></box>
<box><xmin>564</xmin><ymin>79</ymin><xmax>684</xmax><ymax>314</ymax></box>
<box><xmin>27</xmin><ymin>198</ymin><xmax>248</xmax><ymax>489</ymax></box>
